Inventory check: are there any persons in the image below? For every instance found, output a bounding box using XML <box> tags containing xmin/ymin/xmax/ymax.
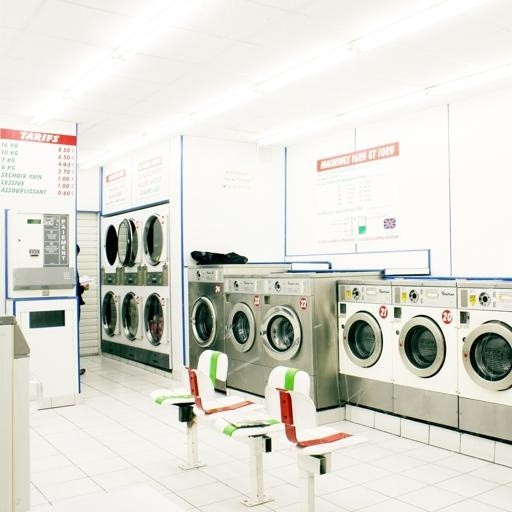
<box><xmin>76</xmin><ymin>243</ymin><xmax>86</xmax><ymax>375</ymax></box>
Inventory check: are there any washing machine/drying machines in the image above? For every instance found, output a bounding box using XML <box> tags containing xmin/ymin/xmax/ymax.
<box><xmin>458</xmin><ymin>283</ymin><xmax>512</xmax><ymax>442</ymax></box>
<box><xmin>99</xmin><ymin>200</ymin><xmax>169</xmax><ymax>370</ymax></box>
<box><xmin>188</xmin><ymin>264</ymin><xmax>291</xmax><ymax>391</ymax></box>
<box><xmin>392</xmin><ymin>281</ymin><xmax>458</xmax><ymax>429</ymax></box>
<box><xmin>223</xmin><ymin>273</ymin><xmax>381</xmax><ymax>408</ymax></box>
<box><xmin>336</xmin><ymin>278</ymin><xmax>394</xmax><ymax>413</ymax></box>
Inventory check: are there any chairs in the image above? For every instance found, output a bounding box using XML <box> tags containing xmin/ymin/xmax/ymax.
<box><xmin>265</xmin><ymin>366</ymin><xmax>371</xmax><ymax>512</ymax></box>
<box><xmin>183</xmin><ymin>366</ymin><xmax>281</xmax><ymax>507</ymax></box>
<box><xmin>219</xmin><ymin>366</ymin><xmax>309</xmax><ymax>507</ymax></box>
<box><xmin>150</xmin><ymin>350</ymin><xmax>228</xmax><ymax>471</ymax></box>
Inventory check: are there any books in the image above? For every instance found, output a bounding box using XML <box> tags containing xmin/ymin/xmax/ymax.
<box><xmin>79</xmin><ymin>275</ymin><xmax>93</xmax><ymax>285</ymax></box>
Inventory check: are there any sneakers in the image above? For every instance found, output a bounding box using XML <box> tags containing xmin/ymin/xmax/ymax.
<box><xmin>80</xmin><ymin>369</ymin><xmax>85</xmax><ymax>374</ymax></box>
